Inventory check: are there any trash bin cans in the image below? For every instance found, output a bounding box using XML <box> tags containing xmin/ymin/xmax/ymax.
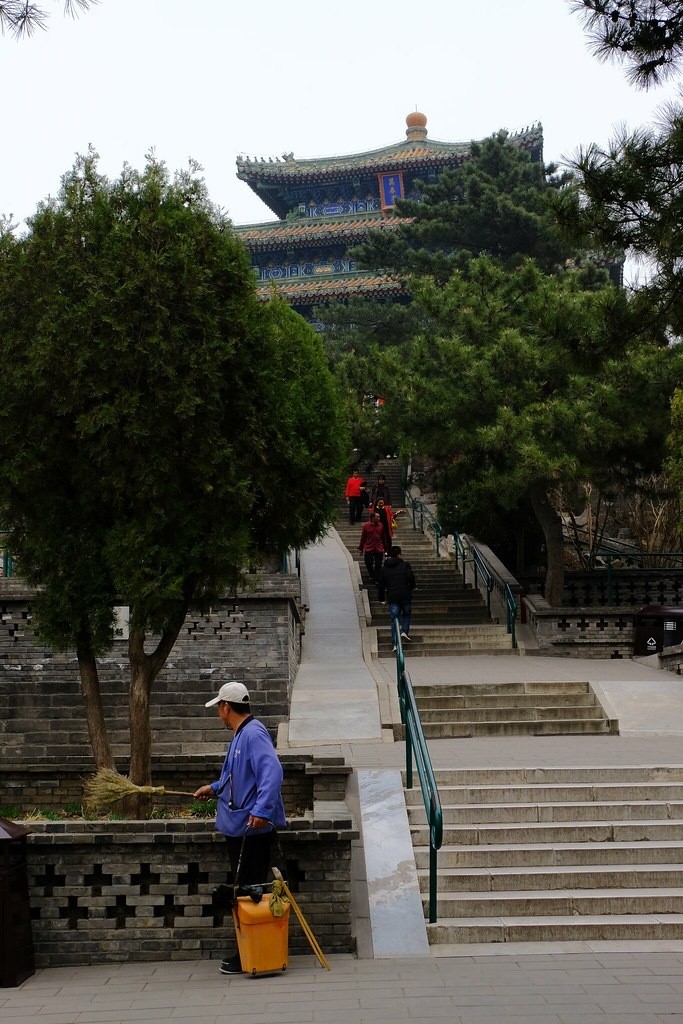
<box><xmin>232</xmin><ymin>893</ymin><xmax>290</xmax><ymax>975</ymax></box>
<box><xmin>0</xmin><ymin>817</ymin><xmax>36</xmax><ymax>988</ymax></box>
<box><xmin>634</xmin><ymin>606</ymin><xmax>666</xmax><ymax>656</ymax></box>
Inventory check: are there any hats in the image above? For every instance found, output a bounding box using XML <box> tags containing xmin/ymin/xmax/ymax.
<box><xmin>205</xmin><ymin>682</ymin><xmax>250</xmax><ymax>707</ymax></box>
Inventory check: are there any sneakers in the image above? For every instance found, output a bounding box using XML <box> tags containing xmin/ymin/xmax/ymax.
<box><xmin>392</xmin><ymin>645</ymin><xmax>397</xmax><ymax>652</ymax></box>
<box><xmin>401</xmin><ymin>633</ymin><xmax>411</xmax><ymax>641</ymax></box>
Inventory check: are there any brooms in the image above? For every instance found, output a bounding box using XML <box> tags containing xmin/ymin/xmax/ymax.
<box><xmin>79</xmin><ymin>765</ymin><xmax>193</xmax><ymax>817</ymax></box>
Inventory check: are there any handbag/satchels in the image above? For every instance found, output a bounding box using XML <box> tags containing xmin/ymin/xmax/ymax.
<box><xmin>391</xmin><ymin>519</ymin><xmax>398</xmax><ymax>528</ymax></box>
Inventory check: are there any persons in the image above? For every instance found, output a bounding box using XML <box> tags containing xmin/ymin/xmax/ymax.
<box><xmin>357</xmin><ymin>474</ymin><xmax>403</xmax><ymax>584</ymax></box>
<box><xmin>379</xmin><ymin>546</ymin><xmax>417</xmax><ymax>652</ymax></box>
<box><xmin>194</xmin><ymin>682</ymin><xmax>287</xmax><ymax>975</ymax></box>
<box><xmin>346</xmin><ymin>469</ymin><xmax>365</xmax><ymax>525</ymax></box>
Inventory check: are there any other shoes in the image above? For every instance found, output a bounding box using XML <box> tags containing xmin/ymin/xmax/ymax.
<box><xmin>219</xmin><ymin>958</ymin><xmax>242</xmax><ymax>973</ymax></box>
